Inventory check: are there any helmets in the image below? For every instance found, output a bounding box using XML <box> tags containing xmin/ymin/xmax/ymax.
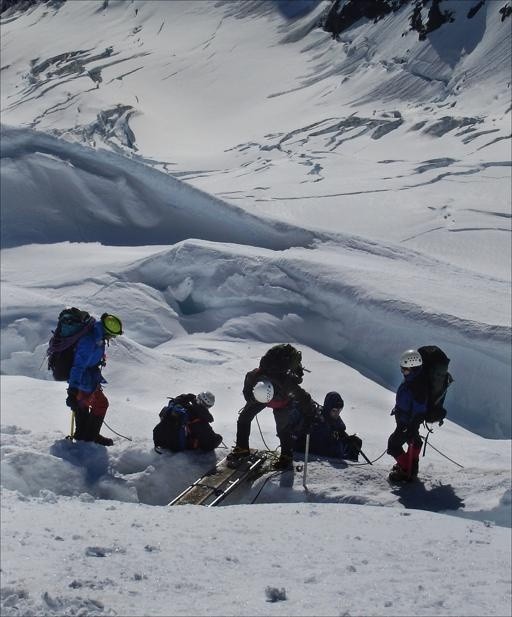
<box><xmin>399</xmin><ymin>349</ymin><xmax>422</xmax><ymax>367</ymax></box>
<box><xmin>102</xmin><ymin>315</ymin><xmax>121</xmax><ymax>336</ymax></box>
<box><xmin>196</xmin><ymin>390</ymin><xmax>215</xmax><ymax>408</ymax></box>
<box><xmin>324</xmin><ymin>391</ymin><xmax>344</xmax><ymax>408</ymax></box>
<box><xmin>252</xmin><ymin>380</ymin><xmax>274</xmax><ymax>403</ymax></box>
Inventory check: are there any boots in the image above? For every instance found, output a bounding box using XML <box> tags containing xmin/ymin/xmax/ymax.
<box><xmin>274</xmin><ymin>454</ymin><xmax>293</xmax><ymax>470</ymax></box>
<box><xmin>389</xmin><ymin>445</ymin><xmax>421</xmax><ymax>481</ymax></box>
<box><xmin>227</xmin><ymin>441</ymin><xmax>250</xmax><ymax>459</ymax></box>
<box><xmin>74</xmin><ymin>407</ymin><xmax>114</xmax><ymax>446</ymax></box>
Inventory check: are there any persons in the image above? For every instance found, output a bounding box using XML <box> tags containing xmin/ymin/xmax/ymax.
<box><xmin>152</xmin><ymin>389</ymin><xmax>222</xmax><ymax>455</ymax></box>
<box><xmin>226</xmin><ymin>366</ymin><xmax>315</xmax><ymax>468</ymax></box>
<box><xmin>386</xmin><ymin>349</ymin><xmax>427</xmax><ymax>483</ymax></box>
<box><xmin>66</xmin><ymin>312</ymin><xmax>124</xmax><ymax>446</ymax></box>
<box><xmin>307</xmin><ymin>390</ymin><xmax>362</xmax><ymax>463</ymax></box>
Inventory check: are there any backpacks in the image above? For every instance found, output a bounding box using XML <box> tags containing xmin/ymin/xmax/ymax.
<box><xmin>46</xmin><ymin>306</ymin><xmax>97</xmax><ymax>381</ymax></box>
<box><xmin>255</xmin><ymin>342</ymin><xmax>304</xmax><ymax>391</ymax></box>
<box><xmin>417</xmin><ymin>344</ymin><xmax>450</xmax><ymax>422</ymax></box>
<box><xmin>153</xmin><ymin>401</ymin><xmax>222</xmax><ymax>451</ymax></box>
